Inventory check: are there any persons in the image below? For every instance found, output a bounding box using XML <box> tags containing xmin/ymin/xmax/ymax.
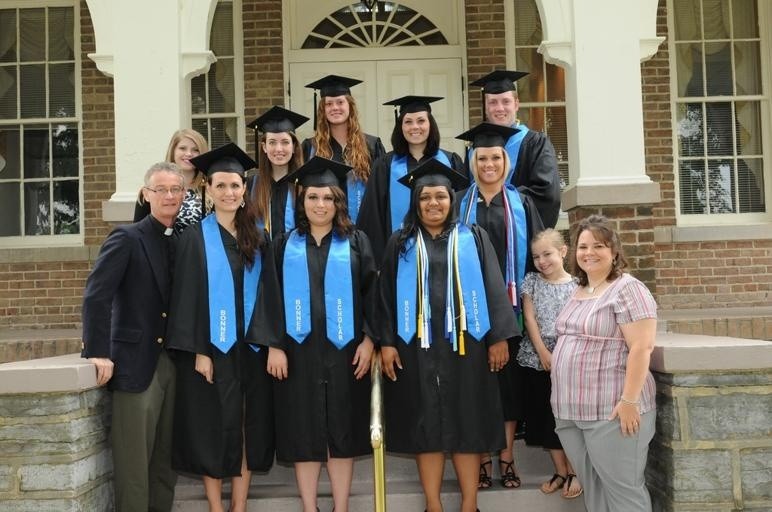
<box><xmin>81</xmin><ymin>122</ymin><xmax>551</xmax><ymax>510</ymax></box>
<box><xmin>546</xmin><ymin>215</ymin><xmax>659</xmax><ymax>511</ymax></box>
<box><xmin>515</xmin><ymin>227</ymin><xmax>584</xmax><ymax>498</ymax></box>
<box><xmin>247</xmin><ymin>71</ymin><xmax>564</xmax><ymax>228</ymax></box>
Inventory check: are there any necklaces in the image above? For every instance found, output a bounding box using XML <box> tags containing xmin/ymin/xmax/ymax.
<box><xmin>586</xmin><ymin>278</ymin><xmax>605</xmax><ymax>294</ymax></box>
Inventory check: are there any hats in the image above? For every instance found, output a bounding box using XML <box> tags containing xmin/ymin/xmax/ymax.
<box><xmin>382</xmin><ymin>96</ymin><xmax>444</xmax><ymax>112</ymax></box>
<box><xmin>282</xmin><ymin>155</ymin><xmax>355</xmax><ymax>186</ymax></box>
<box><xmin>190</xmin><ymin>142</ymin><xmax>258</xmax><ymax>176</ymax></box>
<box><xmin>247</xmin><ymin>106</ymin><xmax>310</xmax><ymax>133</ymax></box>
<box><xmin>397</xmin><ymin>157</ymin><xmax>469</xmax><ymax>192</ymax></box>
<box><xmin>469</xmin><ymin>70</ymin><xmax>530</xmax><ymax>94</ymax></box>
<box><xmin>305</xmin><ymin>75</ymin><xmax>364</xmax><ymax>97</ymax></box>
<box><xmin>454</xmin><ymin>122</ymin><xmax>522</xmax><ymax>149</ymax></box>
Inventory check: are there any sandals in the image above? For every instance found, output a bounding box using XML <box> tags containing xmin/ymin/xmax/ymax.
<box><xmin>540</xmin><ymin>472</ymin><xmax>566</xmax><ymax>493</ymax></box>
<box><xmin>561</xmin><ymin>473</ymin><xmax>583</xmax><ymax>498</ymax></box>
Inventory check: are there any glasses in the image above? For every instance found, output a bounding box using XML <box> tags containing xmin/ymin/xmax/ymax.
<box><xmin>145</xmin><ymin>186</ymin><xmax>185</xmax><ymax>194</ymax></box>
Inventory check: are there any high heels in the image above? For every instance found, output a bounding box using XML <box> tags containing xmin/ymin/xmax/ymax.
<box><xmin>498</xmin><ymin>458</ymin><xmax>521</xmax><ymax>488</ymax></box>
<box><xmin>477</xmin><ymin>460</ymin><xmax>492</xmax><ymax>489</ymax></box>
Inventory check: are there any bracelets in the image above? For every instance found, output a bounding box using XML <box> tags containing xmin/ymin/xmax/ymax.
<box><xmin>620</xmin><ymin>396</ymin><xmax>640</xmax><ymax>405</ymax></box>
